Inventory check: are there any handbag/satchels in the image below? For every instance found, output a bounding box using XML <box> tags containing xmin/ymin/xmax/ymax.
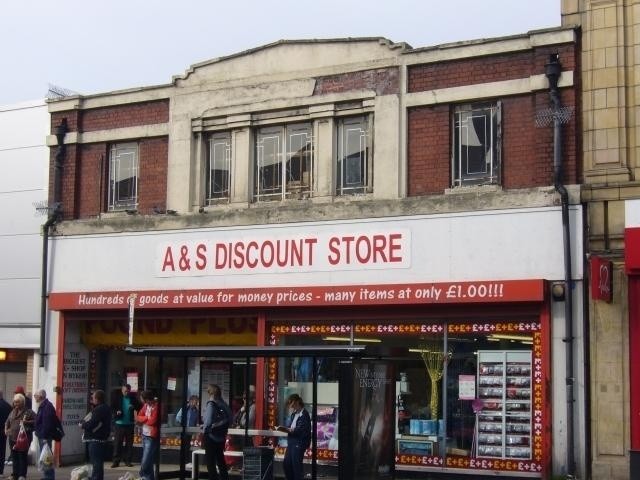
<box><xmin>80</xmin><ymin>411</ymin><xmax>102</xmax><ymax>433</ymax></box>
<box><xmin>206</xmin><ymin>401</ymin><xmax>229</xmax><ymax>432</ymax></box>
<box><xmin>51</xmin><ymin>417</ymin><xmax>64</xmax><ymax>441</ymax></box>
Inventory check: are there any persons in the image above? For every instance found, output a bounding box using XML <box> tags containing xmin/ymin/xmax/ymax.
<box><xmin>0</xmin><ymin>385</ymin><xmax>38</xmax><ymax>480</ymax></box>
<box><xmin>234</xmin><ymin>390</ymin><xmax>256</xmax><ymax>430</ymax></box>
<box><xmin>176</xmin><ymin>395</ymin><xmax>199</xmax><ymax>427</ymax></box>
<box><xmin>33</xmin><ymin>390</ymin><xmax>58</xmax><ymax>479</ymax></box>
<box><xmin>78</xmin><ymin>390</ymin><xmax>111</xmax><ymax>480</ymax></box>
<box><xmin>202</xmin><ymin>384</ymin><xmax>233</xmax><ymax>479</ymax></box>
<box><xmin>135</xmin><ymin>390</ymin><xmax>160</xmax><ymax>480</ymax></box>
<box><xmin>110</xmin><ymin>383</ymin><xmax>141</xmax><ymax>468</ymax></box>
<box><xmin>283</xmin><ymin>393</ymin><xmax>311</xmax><ymax>479</ymax></box>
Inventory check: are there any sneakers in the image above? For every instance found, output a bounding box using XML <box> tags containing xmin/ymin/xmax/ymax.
<box><xmin>125</xmin><ymin>463</ymin><xmax>133</xmax><ymax>467</ymax></box>
<box><xmin>111</xmin><ymin>463</ymin><xmax>119</xmax><ymax>468</ymax></box>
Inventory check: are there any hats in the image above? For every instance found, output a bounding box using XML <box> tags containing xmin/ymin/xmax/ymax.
<box><xmin>15</xmin><ymin>387</ymin><xmax>24</xmax><ymax>393</ymax></box>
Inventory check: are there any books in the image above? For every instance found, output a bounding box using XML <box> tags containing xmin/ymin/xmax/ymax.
<box><xmin>274</xmin><ymin>425</ymin><xmax>290</xmax><ymax>432</ymax></box>
